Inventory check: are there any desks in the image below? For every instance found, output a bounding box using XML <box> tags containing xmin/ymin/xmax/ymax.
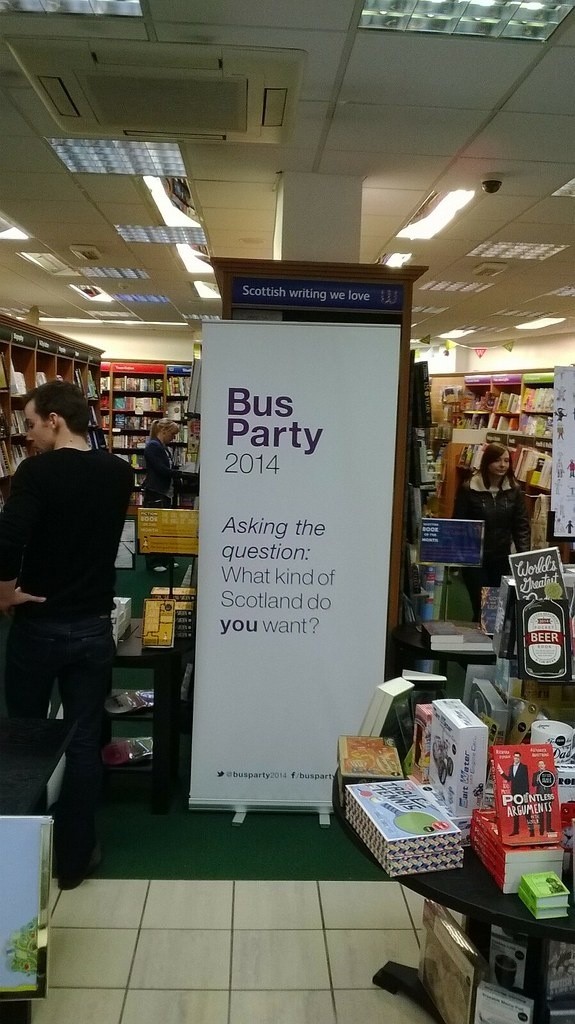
<box><xmin>332</xmin><ymin>770</ymin><xmax>575</xmax><ymax>1024</ymax></box>
<box><xmin>400</xmin><ymin>621</ymin><xmax>495</xmax><ymax>703</ymax></box>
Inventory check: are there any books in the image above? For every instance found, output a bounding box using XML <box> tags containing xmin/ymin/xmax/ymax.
<box><xmin>337</xmin><ymin>623</ymin><xmax>575</xmax><ymax>1023</ymax></box>
<box><xmin>0</xmin><ymin>354</ymin><xmax>199</xmax><ymax>642</ymax></box>
<box><xmin>398</xmin><ymin>362</ymin><xmax>551</xmax><ymax>624</ymax></box>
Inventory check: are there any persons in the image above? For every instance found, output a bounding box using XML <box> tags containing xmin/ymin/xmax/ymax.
<box><xmin>1</xmin><ymin>381</ymin><xmax>133</xmax><ymax>890</ymax></box>
<box><xmin>132</xmin><ymin>421</ymin><xmax>182</xmax><ymax>574</ymax></box>
<box><xmin>454</xmin><ymin>443</ymin><xmax>531</xmax><ymax>622</ymax></box>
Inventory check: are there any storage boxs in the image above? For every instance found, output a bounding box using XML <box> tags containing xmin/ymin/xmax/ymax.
<box><xmin>342</xmin><ymin>674</ymin><xmax>575</xmax><ymax>1024</ymax></box>
<box><xmin>141</xmin><ymin>586</ymin><xmax>195</xmax><ymax>648</ymax></box>
<box><xmin>137</xmin><ymin>507</ymin><xmax>199</xmax><ymax>554</ymax></box>
<box><xmin>111</xmin><ymin>597</ymin><xmax>131</xmax><ymax>645</ymax></box>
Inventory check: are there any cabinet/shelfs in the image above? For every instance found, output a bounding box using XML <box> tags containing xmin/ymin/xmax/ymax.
<box><xmin>429</xmin><ymin>369</ymin><xmax>567</xmax><ymax>564</ymax></box>
<box><xmin>102</xmin><ymin>359</ymin><xmax>201</xmax><ymax>507</ymax></box>
<box><xmin>101</xmin><ymin>618</ymin><xmax>192</xmax><ymax>816</ymax></box>
<box><xmin>2</xmin><ymin>316</ymin><xmax>100</xmax><ymax>479</ymax></box>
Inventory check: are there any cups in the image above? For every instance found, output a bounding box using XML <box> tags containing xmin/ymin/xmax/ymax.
<box><xmin>530</xmin><ymin>720</ymin><xmax>575</xmax><ymax>765</ymax></box>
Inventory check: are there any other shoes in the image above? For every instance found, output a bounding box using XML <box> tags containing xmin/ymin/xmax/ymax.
<box><xmin>57</xmin><ymin>841</ymin><xmax>100</xmax><ymax>890</ymax></box>
<box><xmin>174</xmin><ymin>562</ymin><xmax>179</xmax><ymax>568</ymax></box>
<box><xmin>153</xmin><ymin>566</ymin><xmax>167</xmax><ymax>572</ymax></box>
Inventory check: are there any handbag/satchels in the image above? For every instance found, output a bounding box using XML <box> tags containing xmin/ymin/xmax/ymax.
<box><xmin>173</xmin><ymin>471</ymin><xmax>199</xmax><ymax>493</ymax></box>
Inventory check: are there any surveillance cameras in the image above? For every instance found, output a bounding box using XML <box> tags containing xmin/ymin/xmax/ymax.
<box><xmin>481</xmin><ymin>172</ymin><xmax>504</xmax><ymax>193</ymax></box>
